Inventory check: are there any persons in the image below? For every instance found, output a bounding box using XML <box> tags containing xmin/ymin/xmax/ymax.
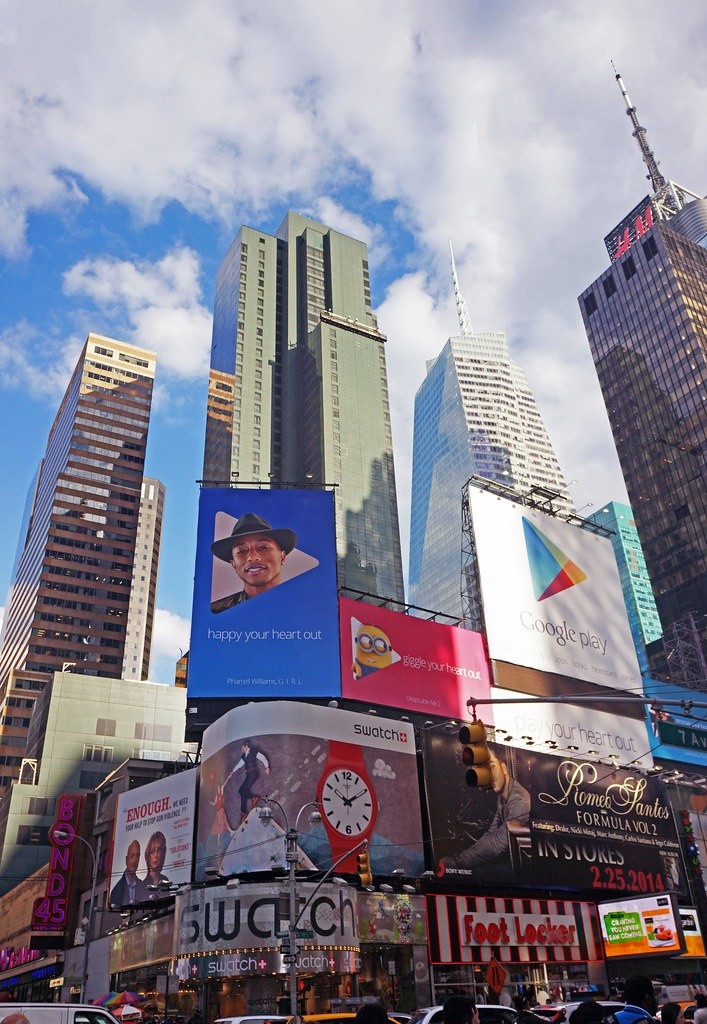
<box><xmin>229</xmin><ymin>742</ymin><xmax>270</xmax><ymax>826</ymax></box>
<box><xmin>367</xmin><ymin>900</ymin><xmax>415</xmax><ymax>944</ymax></box>
<box><xmin>442</xmin><ymin>743</ymin><xmax>532</xmax><ymax>868</ymax></box>
<box><xmin>211</xmin><ymin>513</ymin><xmax>297</xmax><ymax>614</ymax></box>
<box><xmin>356</xmin><ymin>1005</ymin><xmax>388</xmax><ymax>1024</ymax></box>
<box><xmin>110</xmin><ymin>831</ymin><xmax>170</xmax><ymax>905</ymax></box>
<box><xmin>209</xmin><ymin>774</ymin><xmax>237</xmax><ymax>846</ymax></box>
<box><xmin>511</xmin><ymin>986</ymin><xmax>566</xmax><ymax>1024</ymax></box>
<box><xmin>443</xmin><ymin>995</ymin><xmax>480</xmax><ymax>1024</ymax></box>
<box><xmin>651</xmin><ymin>704</ymin><xmax>674</xmax><ymax>723</ymax></box>
<box><xmin>569</xmin><ymin>975</ymin><xmax>707</xmax><ymax>1024</ymax></box>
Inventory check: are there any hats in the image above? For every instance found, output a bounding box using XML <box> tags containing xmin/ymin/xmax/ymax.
<box><xmin>212</xmin><ymin>511</ymin><xmax>297</xmax><ymax>564</ymax></box>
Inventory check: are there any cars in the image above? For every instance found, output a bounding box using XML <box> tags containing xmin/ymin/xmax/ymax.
<box><xmin>211</xmin><ymin>1000</ymin><xmax>699</xmax><ymax>1024</ymax></box>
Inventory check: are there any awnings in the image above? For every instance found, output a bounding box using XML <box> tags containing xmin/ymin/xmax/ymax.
<box><xmin>331</xmin><ymin>996</ymin><xmax>377</xmax><ymax>1006</ymax></box>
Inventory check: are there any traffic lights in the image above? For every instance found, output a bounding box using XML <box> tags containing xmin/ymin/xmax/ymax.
<box><xmin>459</xmin><ymin>719</ymin><xmax>495</xmax><ymax>792</ymax></box>
<box><xmin>356</xmin><ymin>851</ymin><xmax>372</xmax><ymax>885</ymax></box>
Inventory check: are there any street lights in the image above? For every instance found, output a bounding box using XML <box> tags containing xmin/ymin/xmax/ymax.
<box><xmin>53</xmin><ymin>830</ymin><xmax>102</xmax><ymax>1004</ymax></box>
<box><xmin>264</xmin><ymin>794</ymin><xmax>323</xmax><ymax>1015</ymax></box>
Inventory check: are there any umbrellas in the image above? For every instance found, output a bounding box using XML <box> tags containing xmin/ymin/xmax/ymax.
<box><xmin>91</xmin><ymin>990</ymin><xmax>149</xmax><ymax>1016</ymax></box>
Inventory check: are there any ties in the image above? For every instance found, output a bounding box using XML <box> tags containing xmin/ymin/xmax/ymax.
<box><xmin>502</xmin><ymin>800</ymin><xmax>508</xmax><ymax>819</ymax></box>
<box><xmin>130</xmin><ymin>886</ymin><xmax>134</xmax><ymax>898</ymax></box>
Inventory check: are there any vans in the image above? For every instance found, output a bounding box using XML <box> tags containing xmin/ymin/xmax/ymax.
<box><xmin>0</xmin><ymin>1002</ymin><xmax>123</xmax><ymax>1024</ymax></box>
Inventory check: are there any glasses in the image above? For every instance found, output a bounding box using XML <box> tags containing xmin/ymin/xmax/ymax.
<box><xmin>149</xmin><ymin>846</ymin><xmax>164</xmax><ymax>856</ymax></box>
<box><xmin>354</xmin><ymin>633</ymin><xmax>391</xmax><ymax>656</ymax></box>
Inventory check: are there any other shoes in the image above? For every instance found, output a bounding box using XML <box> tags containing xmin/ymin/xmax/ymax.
<box><xmin>238</xmin><ymin>811</ymin><xmax>249</xmax><ymax>824</ymax></box>
<box><xmin>230</xmin><ymin>828</ymin><xmax>238</xmax><ymax>837</ymax></box>
<box><xmin>250</xmin><ymin>795</ymin><xmax>259</xmax><ymax>810</ymax></box>
